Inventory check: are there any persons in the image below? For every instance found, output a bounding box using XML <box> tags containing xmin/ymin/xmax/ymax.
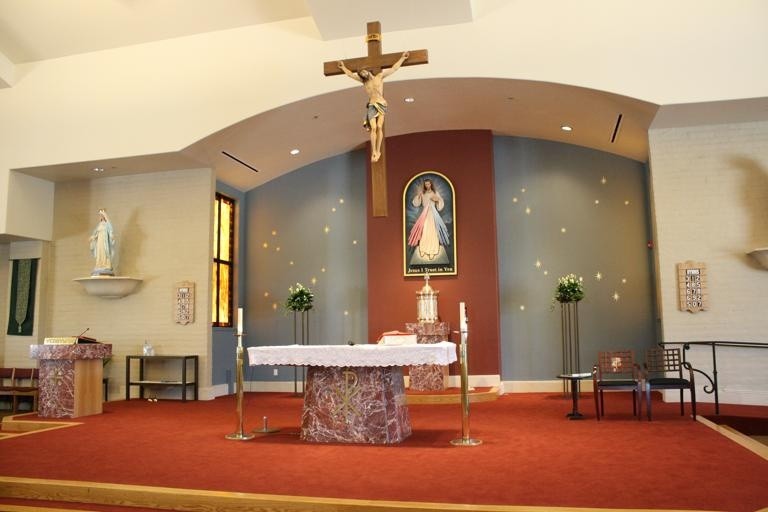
<box><xmin>406</xmin><ymin>179</ymin><xmax>452</xmax><ymax>260</ymax></box>
<box><xmin>87</xmin><ymin>207</ymin><xmax>115</xmax><ymax>276</ymax></box>
<box><xmin>337</xmin><ymin>51</ymin><xmax>411</xmax><ymax>167</ymax></box>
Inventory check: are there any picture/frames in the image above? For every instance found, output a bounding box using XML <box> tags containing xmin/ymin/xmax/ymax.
<box><xmin>402</xmin><ymin>171</ymin><xmax>458</xmax><ymax>278</ymax></box>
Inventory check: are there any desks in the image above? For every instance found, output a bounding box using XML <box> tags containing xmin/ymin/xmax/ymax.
<box><xmin>556</xmin><ymin>374</ymin><xmax>592</xmax><ymax>420</ymax></box>
<box><xmin>247</xmin><ymin>344</ymin><xmax>457</xmax><ymax>444</ymax></box>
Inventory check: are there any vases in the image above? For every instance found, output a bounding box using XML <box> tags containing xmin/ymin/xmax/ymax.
<box><xmin>556</xmin><ymin>297</ymin><xmax>582</xmax><ymax>302</ymax></box>
<box><xmin>291</xmin><ymin>306</ymin><xmax>312</xmax><ymax>311</ymax></box>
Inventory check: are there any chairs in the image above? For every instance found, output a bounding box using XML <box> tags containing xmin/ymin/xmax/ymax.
<box><xmin>0</xmin><ymin>368</ymin><xmax>39</xmax><ymax>415</ymax></box>
<box><xmin>592</xmin><ymin>348</ymin><xmax>696</xmax><ymax>421</ymax></box>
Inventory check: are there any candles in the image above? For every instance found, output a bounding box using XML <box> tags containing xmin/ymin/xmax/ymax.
<box><xmin>237</xmin><ymin>307</ymin><xmax>243</xmax><ymax>334</ymax></box>
<box><xmin>459</xmin><ymin>302</ymin><xmax>466</xmax><ymax>331</ymax></box>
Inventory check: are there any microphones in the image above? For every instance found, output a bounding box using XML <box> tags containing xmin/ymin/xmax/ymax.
<box><xmin>78</xmin><ymin>327</ymin><xmax>89</xmax><ymax>338</ymax></box>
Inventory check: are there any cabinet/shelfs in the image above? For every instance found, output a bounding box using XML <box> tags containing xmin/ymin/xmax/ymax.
<box><xmin>125</xmin><ymin>355</ymin><xmax>199</xmax><ymax>403</ymax></box>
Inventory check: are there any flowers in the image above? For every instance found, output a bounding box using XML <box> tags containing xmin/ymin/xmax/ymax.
<box><xmin>549</xmin><ymin>272</ymin><xmax>588</xmax><ymax>312</ymax></box>
<box><xmin>280</xmin><ymin>281</ymin><xmax>314</xmax><ymax>317</ymax></box>
